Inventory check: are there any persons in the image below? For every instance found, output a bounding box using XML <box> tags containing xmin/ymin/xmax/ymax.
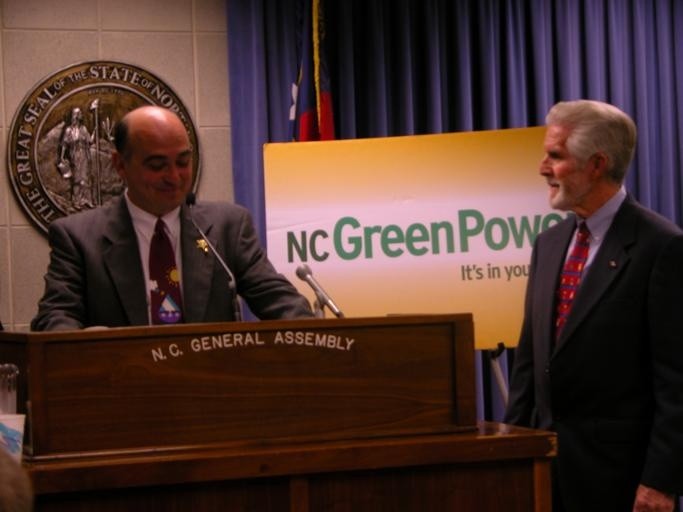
<box><xmin>500</xmin><ymin>99</ymin><xmax>683</xmax><ymax>512</ymax></box>
<box><xmin>59</xmin><ymin>107</ymin><xmax>97</xmax><ymax>212</ymax></box>
<box><xmin>30</xmin><ymin>105</ymin><xmax>314</xmax><ymax>333</ymax></box>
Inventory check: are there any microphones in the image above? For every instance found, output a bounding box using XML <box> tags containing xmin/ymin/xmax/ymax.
<box><xmin>295</xmin><ymin>264</ymin><xmax>345</xmax><ymax>318</ymax></box>
<box><xmin>185</xmin><ymin>193</ymin><xmax>243</xmax><ymax>322</ymax></box>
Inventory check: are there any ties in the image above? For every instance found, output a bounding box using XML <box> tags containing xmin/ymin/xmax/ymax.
<box><xmin>147</xmin><ymin>219</ymin><xmax>185</xmax><ymax>325</ymax></box>
<box><xmin>549</xmin><ymin>221</ymin><xmax>591</xmax><ymax>351</ymax></box>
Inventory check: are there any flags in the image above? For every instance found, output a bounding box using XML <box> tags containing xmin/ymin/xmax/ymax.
<box><xmin>287</xmin><ymin>0</ymin><xmax>336</xmax><ymax>142</ymax></box>
